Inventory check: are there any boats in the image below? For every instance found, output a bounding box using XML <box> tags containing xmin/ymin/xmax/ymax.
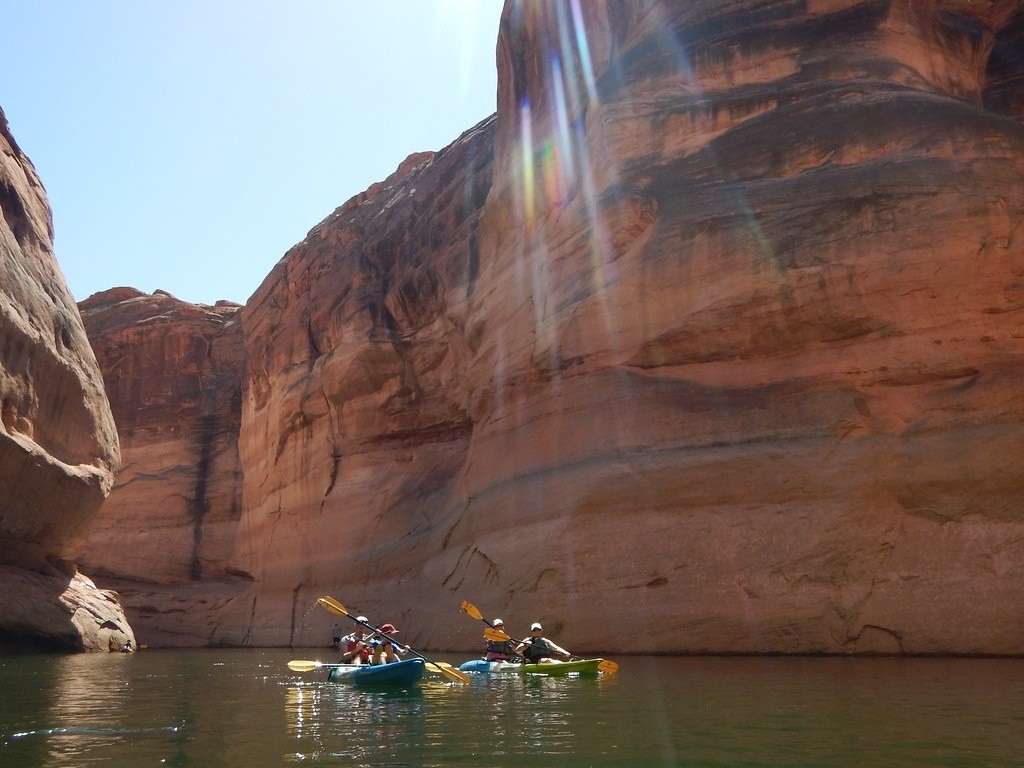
<box><xmin>327</xmin><ymin>656</ymin><xmax>426</xmax><ymax>687</ymax></box>
<box><xmin>459</xmin><ymin>656</ymin><xmax>619</xmax><ymax>680</ymax></box>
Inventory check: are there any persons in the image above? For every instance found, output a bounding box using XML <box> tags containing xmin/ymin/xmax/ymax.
<box><xmin>482</xmin><ymin>618</ymin><xmax>517</xmax><ymax>663</ymax></box>
<box><xmin>363</xmin><ymin>624</ymin><xmax>411</xmax><ymax>666</ymax></box>
<box><xmin>339</xmin><ymin>614</ymin><xmax>376</xmax><ymax>666</ymax></box>
<box><xmin>515</xmin><ymin>621</ymin><xmax>572</xmax><ymax>664</ymax></box>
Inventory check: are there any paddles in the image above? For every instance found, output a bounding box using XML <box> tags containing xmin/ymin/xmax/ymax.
<box><xmin>484</xmin><ymin>627</ymin><xmax>620</xmax><ymax>675</ymax></box>
<box><xmin>462</xmin><ymin>601</ymin><xmax>518</xmax><ymax>648</ymax></box>
<box><xmin>318</xmin><ymin>595</ymin><xmax>470</xmax><ymax>685</ymax></box>
<box><xmin>288</xmin><ymin>659</ymin><xmax>453</xmax><ymax>673</ymax></box>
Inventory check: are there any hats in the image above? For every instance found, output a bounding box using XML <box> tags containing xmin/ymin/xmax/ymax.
<box><xmin>530</xmin><ymin>622</ymin><xmax>542</xmax><ymax>631</ymax></box>
<box><xmin>493</xmin><ymin>618</ymin><xmax>503</xmax><ymax>626</ymax></box>
<box><xmin>380</xmin><ymin>624</ymin><xmax>399</xmax><ymax>636</ymax></box>
